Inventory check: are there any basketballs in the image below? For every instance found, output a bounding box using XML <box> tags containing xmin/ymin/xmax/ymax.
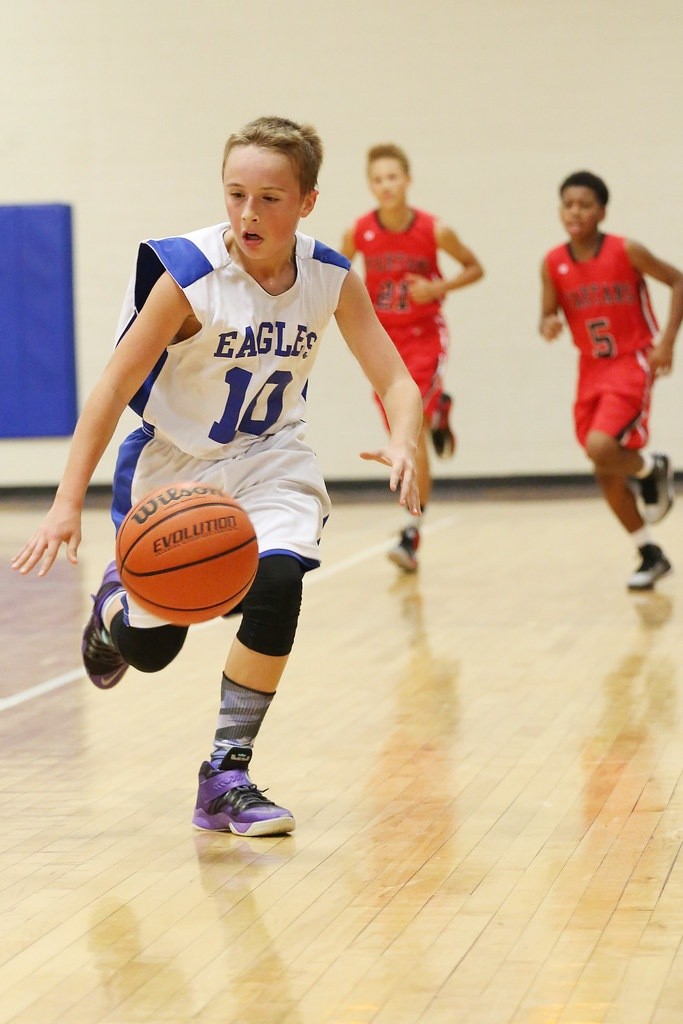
<box><xmin>115</xmin><ymin>483</ymin><xmax>261</xmax><ymax>622</ymax></box>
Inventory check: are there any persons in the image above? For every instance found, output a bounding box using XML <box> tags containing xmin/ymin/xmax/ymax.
<box><xmin>539</xmin><ymin>172</ymin><xmax>682</xmax><ymax>592</ymax></box>
<box><xmin>342</xmin><ymin>144</ymin><xmax>483</xmax><ymax>572</ymax></box>
<box><xmin>9</xmin><ymin>117</ymin><xmax>423</xmax><ymax>837</ymax></box>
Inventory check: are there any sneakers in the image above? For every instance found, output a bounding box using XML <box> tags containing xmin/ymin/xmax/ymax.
<box><xmin>191</xmin><ymin>745</ymin><xmax>296</xmax><ymax>838</ymax></box>
<box><xmin>387</xmin><ymin>525</ymin><xmax>421</xmax><ymax>574</ymax></box>
<box><xmin>626</xmin><ymin>545</ymin><xmax>672</xmax><ymax>589</ymax></box>
<box><xmin>81</xmin><ymin>557</ymin><xmax>128</xmax><ymax>692</ymax></box>
<box><xmin>638</xmin><ymin>454</ymin><xmax>673</xmax><ymax>523</ymax></box>
<box><xmin>430</xmin><ymin>393</ymin><xmax>455</xmax><ymax>461</ymax></box>
